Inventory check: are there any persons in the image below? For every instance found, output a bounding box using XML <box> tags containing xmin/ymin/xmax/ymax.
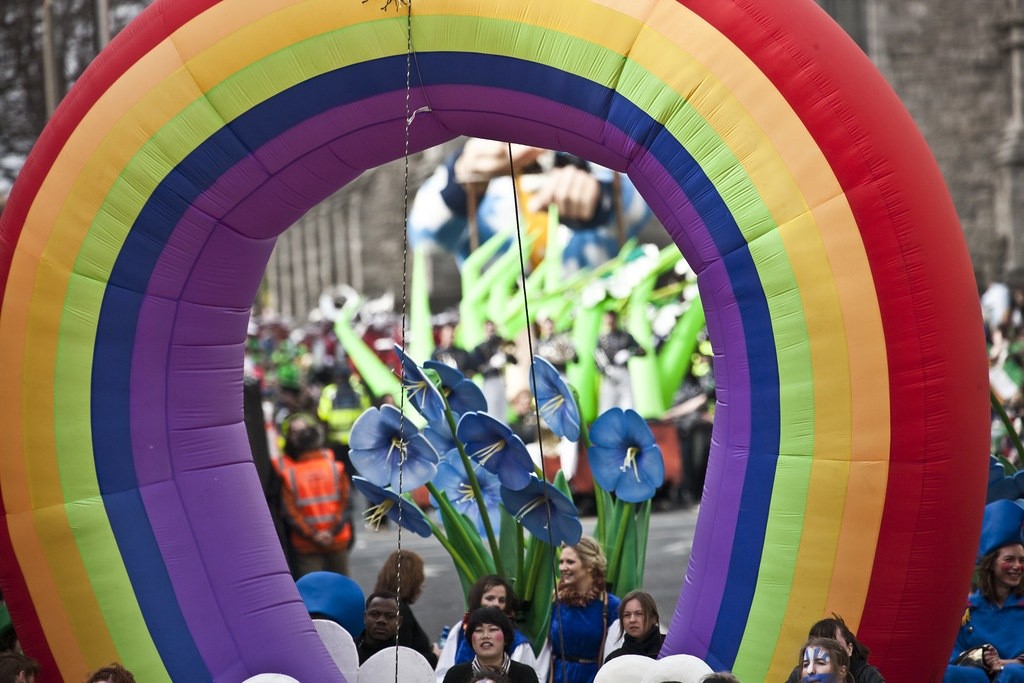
<box><xmin>537</xmin><ymin>535</ymin><xmax>622</xmax><ymax>683</ymax></box>
<box><xmin>407</xmin><ymin>136</ymin><xmax>649</xmax><ymax>286</ymax></box>
<box><xmin>443</xmin><ymin>607</ymin><xmax>539</xmax><ymax>683</ymax></box>
<box><xmin>604</xmin><ymin>591</ymin><xmax>667</xmax><ymax>664</ymax></box>
<box><xmin>0</xmin><ymin>272</ymin><xmax>1024</xmax><ymax>683</ymax></box>
<box><xmin>428</xmin><ymin>574</ymin><xmax>537</xmax><ymax>683</ymax></box>
